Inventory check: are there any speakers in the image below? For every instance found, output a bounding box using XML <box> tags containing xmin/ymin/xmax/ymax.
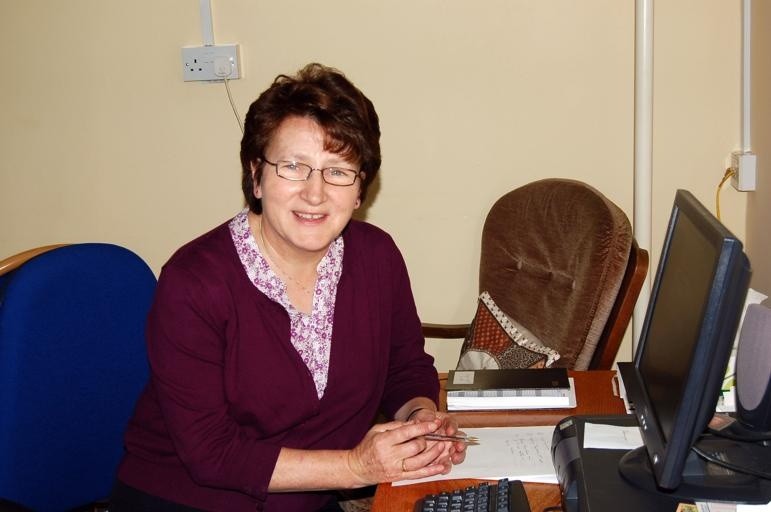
<box><xmin>733</xmin><ymin>302</ymin><xmax>771</xmax><ymax>441</ymax></box>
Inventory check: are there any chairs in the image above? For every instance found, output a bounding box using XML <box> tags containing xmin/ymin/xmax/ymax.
<box><xmin>420</xmin><ymin>178</ymin><xmax>650</xmax><ymax>373</ymax></box>
<box><xmin>1</xmin><ymin>243</ymin><xmax>159</xmax><ymax>510</ymax></box>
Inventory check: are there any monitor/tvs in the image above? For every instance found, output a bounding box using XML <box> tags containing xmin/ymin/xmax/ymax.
<box><xmin>617</xmin><ymin>187</ymin><xmax>770</xmax><ymax>495</ymax></box>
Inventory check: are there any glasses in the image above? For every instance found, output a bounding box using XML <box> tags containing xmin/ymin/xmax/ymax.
<box><xmin>264</xmin><ymin>157</ymin><xmax>361</xmax><ymax>186</ymax></box>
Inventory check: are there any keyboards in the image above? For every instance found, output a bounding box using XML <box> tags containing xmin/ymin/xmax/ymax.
<box><xmin>414</xmin><ymin>477</ymin><xmax>532</xmax><ymax>511</ymax></box>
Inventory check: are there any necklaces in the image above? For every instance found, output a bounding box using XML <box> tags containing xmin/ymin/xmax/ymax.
<box><xmin>261</xmin><ymin>220</ymin><xmax>313</xmax><ymax>295</ymax></box>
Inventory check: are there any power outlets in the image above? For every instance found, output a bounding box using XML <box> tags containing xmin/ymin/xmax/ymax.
<box><xmin>181</xmin><ymin>44</ymin><xmax>240</xmax><ymax>82</ymax></box>
<box><xmin>730</xmin><ymin>151</ymin><xmax>758</xmax><ymax>192</ymax></box>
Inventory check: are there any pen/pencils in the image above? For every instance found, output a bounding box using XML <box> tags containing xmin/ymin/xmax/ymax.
<box><xmin>372</xmin><ymin>427</ymin><xmax>479</xmax><ymax>446</ymax></box>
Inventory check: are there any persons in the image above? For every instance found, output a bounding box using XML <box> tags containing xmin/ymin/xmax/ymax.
<box><xmin>109</xmin><ymin>63</ymin><xmax>467</xmax><ymax>512</ymax></box>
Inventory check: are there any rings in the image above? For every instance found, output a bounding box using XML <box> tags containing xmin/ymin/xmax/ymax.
<box><xmin>402</xmin><ymin>459</ymin><xmax>408</xmax><ymax>472</ymax></box>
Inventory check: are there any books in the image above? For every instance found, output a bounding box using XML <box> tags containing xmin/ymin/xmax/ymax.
<box><xmin>444</xmin><ymin>367</ymin><xmax>570</xmax><ymax>413</ymax></box>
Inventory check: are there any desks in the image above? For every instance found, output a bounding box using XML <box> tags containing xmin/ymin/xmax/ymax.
<box><xmin>372</xmin><ymin>369</ymin><xmax>629</xmax><ymax>512</ymax></box>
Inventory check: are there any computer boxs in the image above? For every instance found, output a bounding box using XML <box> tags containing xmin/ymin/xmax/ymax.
<box><xmin>549</xmin><ymin>411</ymin><xmax>770</xmax><ymax>512</ymax></box>
